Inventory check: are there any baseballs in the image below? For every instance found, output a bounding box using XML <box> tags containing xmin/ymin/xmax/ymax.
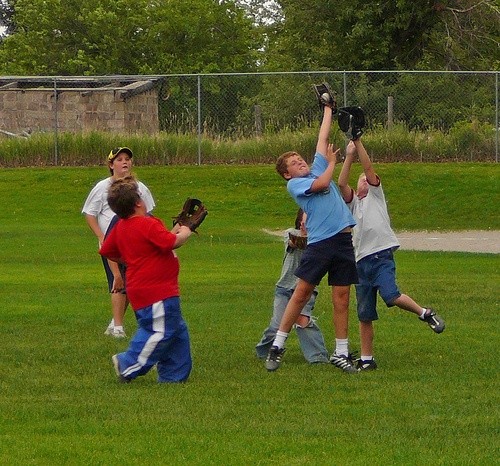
<box><xmin>321</xmin><ymin>93</ymin><xmax>330</xmax><ymax>102</ymax></box>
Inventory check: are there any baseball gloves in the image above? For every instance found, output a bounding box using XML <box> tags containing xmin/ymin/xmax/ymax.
<box><xmin>172</xmin><ymin>196</ymin><xmax>208</xmax><ymax>234</ymax></box>
<box><xmin>312</xmin><ymin>81</ymin><xmax>337</xmax><ymax>116</ymax></box>
<box><xmin>338</xmin><ymin>106</ymin><xmax>365</xmax><ymax>141</ymax></box>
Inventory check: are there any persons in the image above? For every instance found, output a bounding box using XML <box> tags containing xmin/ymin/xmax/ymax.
<box><xmin>102</xmin><ymin>174</ymin><xmax>154</xmax><ymax>294</ymax></box>
<box><xmin>264</xmin><ymin>91</ymin><xmax>358</xmax><ymax>375</ymax></box>
<box><xmin>256</xmin><ymin>208</ymin><xmax>331</xmax><ymax>364</ymax></box>
<box><xmin>82</xmin><ymin>146</ymin><xmax>156</xmax><ymax>340</ymax></box>
<box><xmin>99</xmin><ymin>182</ymin><xmax>208</xmax><ymax>384</ymax></box>
<box><xmin>337</xmin><ymin>106</ymin><xmax>446</xmax><ymax>371</ymax></box>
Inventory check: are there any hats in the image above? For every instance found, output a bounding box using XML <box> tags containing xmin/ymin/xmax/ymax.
<box><xmin>107</xmin><ymin>147</ymin><xmax>133</xmax><ymax>163</ymax></box>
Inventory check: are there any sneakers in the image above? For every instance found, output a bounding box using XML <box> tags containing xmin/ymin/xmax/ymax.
<box><xmin>111</xmin><ymin>354</ymin><xmax>130</xmax><ymax>384</ymax></box>
<box><xmin>111</xmin><ymin>329</ymin><xmax>126</xmax><ymax>339</ymax></box>
<box><xmin>104</xmin><ymin>327</ymin><xmax>113</xmax><ymax>336</ymax></box>
<box><xmin>265</xmin><ymin>345</ymin><xmax>287</xmax><ymax>371</ymax></box>
<box><xmin>329</xmin><ymin>350</ymin><xmax>358</xmax><ymax>374</ymax></box>
<box><xmin>353</xmin><ymin>359</ymin><xmax>377</xmax><ymax>373</ymax></box>
<box><xmin>418</xmin><ymin>307</ymin><xmax>445</xmax><ymax>333</ymax></box>
